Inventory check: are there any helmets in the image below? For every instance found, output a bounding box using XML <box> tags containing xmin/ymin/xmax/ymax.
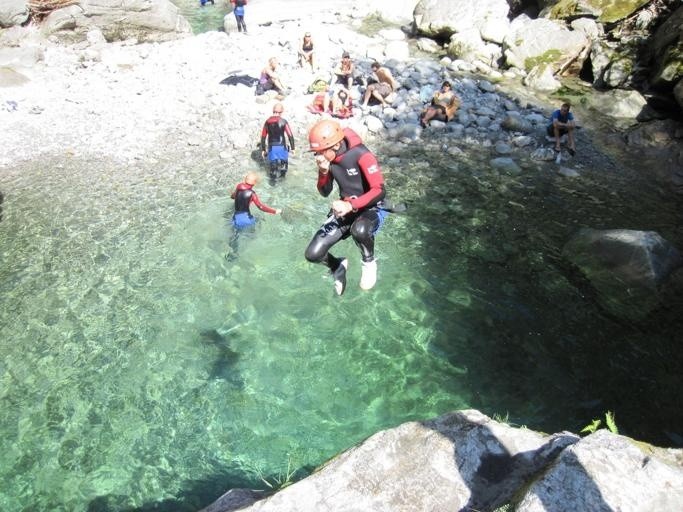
<box><xmin>273</xmin><ymin>103</ymin><xmax>284</xmax><ymax>113</ymax></box>
<box><xmin>309</xmin><ymin>118</ymin><xmax>345</xmax><ymax>151</ymax></box>
<box><xmin>245</xmin><ymin>171</ymin><xmax>257</xmax><ymax>185</ymax></box>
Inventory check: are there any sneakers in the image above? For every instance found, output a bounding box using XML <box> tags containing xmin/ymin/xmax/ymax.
<box><xmin>332</xmin><ymin>256</ymin><xmax>347</xmax><ymax>296</ymax></box>
<box><xmin>360</xmin><ymin>255</ymin><xmax>377</xmax><ymax>290</ymax></box>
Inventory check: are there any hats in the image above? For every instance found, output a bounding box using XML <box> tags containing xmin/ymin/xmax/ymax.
<box><xmin>342</xmin><ymin>52</ymin><xmax>350</xmax><ymax>58</ymax></box>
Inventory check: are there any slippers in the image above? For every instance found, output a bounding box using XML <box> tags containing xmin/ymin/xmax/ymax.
<box><xmin>555</xmin><ymin>145</ymin><xmax>577</xmax><ymax>154</ymax></box>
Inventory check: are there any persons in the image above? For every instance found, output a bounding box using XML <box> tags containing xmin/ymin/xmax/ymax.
<box><xmin>328</xmin><ymin>49</ymin><xmax>355</xmax><ymax>89</ymax></box>
<box><xmin>356</xmin><ymin>60</ymin><xmax>396</xmax><ymax>112</ymax></box>
<box><xmin>296</xmin><ymin>29</ymin><xmax>319</xmax><ymax>73</ymax></box>
<box><xmin>543</xmin><ymin>102</ymin><xmax>578</xmax><ymax>154</ymax></box>
<box><xmin>419</xmin><ymin>79</ymin><xmax>461</xmax><ymax>129</ymax></box>
<box><xmin>320</xmin><ymin>81</ymin><xmax>350</xmax><ymax>116</ymax></box>
<box><xmin>300</xmin><ymin>117</ymin><xmax>389</xmax><ymax>299</ymax></box>
<box><xmin>224</xmin><ymin>171</ymin><xmax>282</xmax><ymax>265</ymax></box>
<box><xmin>257</xmin><ymin>55</ymin><xmax>292</xmax><ymax>98</ymax></box>
<box><xmin>257</xmin><ymin>101</ymin><xmax>297</xmax><ymax>186</ymax></box>
<box><xmin>228</xmin><ymin>0</ymin><xmax>249</xmax><ymax>33</ymax></box>
